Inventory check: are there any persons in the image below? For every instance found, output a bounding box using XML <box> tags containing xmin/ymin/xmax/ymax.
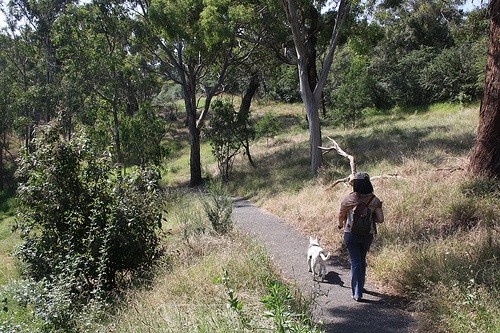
<box><xmin>338</xmin><ymin>172</ymin><xmax>384</xmax><ymax>302</ymax></box>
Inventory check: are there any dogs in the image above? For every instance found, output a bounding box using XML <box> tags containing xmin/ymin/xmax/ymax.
<box><xmin>307</xmin><ymin>235</ymin><xmax>331</xmax><ymax>284</ymax></box>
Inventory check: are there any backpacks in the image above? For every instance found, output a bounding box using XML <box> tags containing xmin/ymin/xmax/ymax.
<box><xmin>352</xmin><ymin>203</ymin><xmax>371</xmax><ymax>238</ymax></box>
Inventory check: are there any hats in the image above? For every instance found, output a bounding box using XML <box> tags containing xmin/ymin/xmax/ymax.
<box><xmin>350</xmin><ymin>171</ymin><xmax>370</xmax><ymax>186</ymax></box>
